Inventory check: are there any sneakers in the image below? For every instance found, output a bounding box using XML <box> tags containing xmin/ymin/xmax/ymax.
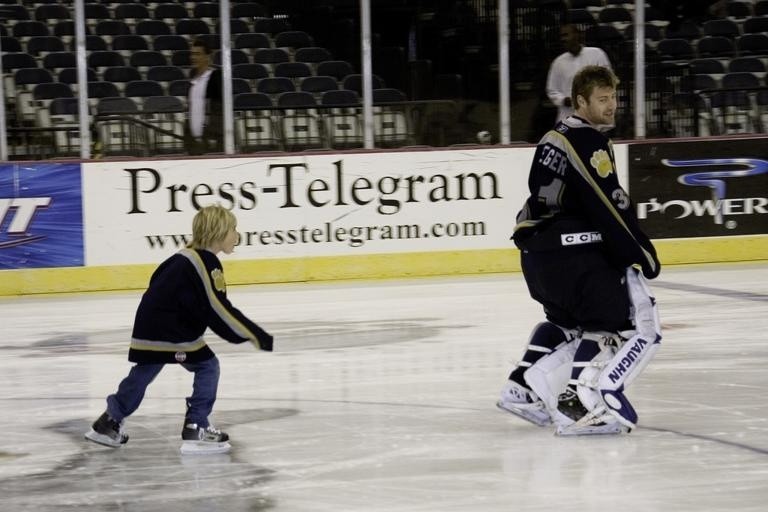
<box><xmin>503</xmin><ymin>381</ymin><xmax>574</xmax><ymax>422</ymax></box>
<box><xmin>182</xmin><ymin>416</ymin><xmax>229</xmax><ymax>442</ymax></box>
<box><xmin>558</xmin><ymin>388</ymin><xmax>618</xmax><ymax>426</ymax></box>
<box><xmin>92</xmin><ymin>409</ymin><xmax>128</xmax><ymax>444</ymax></box>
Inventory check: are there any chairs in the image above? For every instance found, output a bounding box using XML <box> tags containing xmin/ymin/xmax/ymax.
<box><xmin>1</xmin><ymin>0</ymin><xmax>768</xmax><ymax>157</ymax></box>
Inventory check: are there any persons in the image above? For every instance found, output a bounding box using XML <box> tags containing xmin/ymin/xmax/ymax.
<box><xmin>92</xmin><ymin>204</ymin><xmax>273</xmax><ymax>443</ymax></box>
<box><xmin>183</xmin><ymin>41</ymin><xmax>224</xmax><ymax>155</ymax></box>
<box><xmin>662</xmin><ymin>0</ymin><xmax>729</xmax><ymax>29</ymax></box>
<box><xmin>407</xmin><ymin>15</ymin><xmax>433</xmax><ymax>98</ymax></box>
